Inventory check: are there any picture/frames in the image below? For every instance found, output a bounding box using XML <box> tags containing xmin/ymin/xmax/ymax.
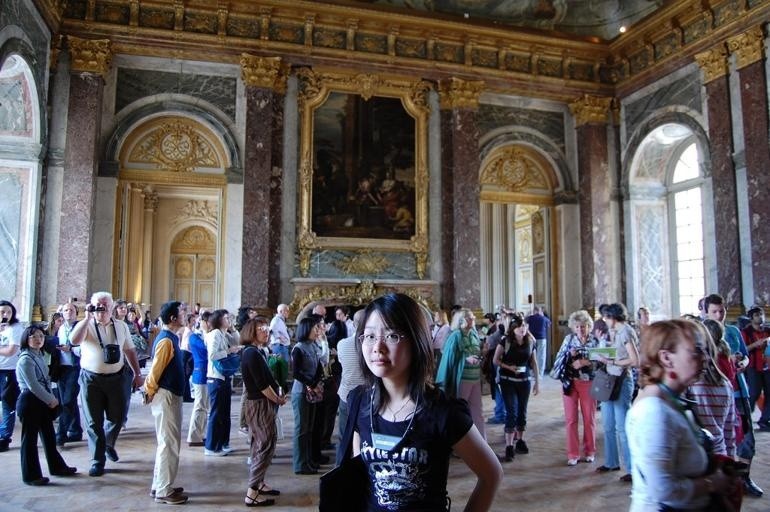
<box><xmin>288</xmin><ymin>57</ymin><xmax>435</xmax><ymax>287</ymax></box>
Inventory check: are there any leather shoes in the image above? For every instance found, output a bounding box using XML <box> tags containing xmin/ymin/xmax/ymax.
<box><xmin>89</xmin><ymin>464</ymin><xmax>104</xmax><ymax>476</ymax></box>
<box><xmin>745</xmin><ymin>477</ymin><xmax>763</xmax><ymax>497</ymax></box>
<box><xmin>105</xmin><ymin>449</ymin><xmax>118</xmax><ymax>462</ymax></box>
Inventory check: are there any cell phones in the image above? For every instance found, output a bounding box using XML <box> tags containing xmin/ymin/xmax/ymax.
<box><xmin>1</xmin><ymin>318</ymin><xmax>8</xmax><ymax>323</ymax></box>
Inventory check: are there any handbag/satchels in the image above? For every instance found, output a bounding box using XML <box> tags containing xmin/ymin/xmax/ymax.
<box><xmin>319</xmin><ymin>453</ymin><xmax>369</xmax><ymax>512</ymax></box>
<box><xmin>267</xmin><ymin>355</ymin><xmax>288</xmax><ymax>383</ymax></box>
<box><xmin>131</xmin><ymin>335</ymin><xmax>147</xmax><ymax>350</ymax></box>
<box><xmin>50</xmin><ymin>389</ymin><xmax>63</xmax><ymax>420</ymax></box>
<box><xmin>212</xmin><ymin>353</ymin><xmax>240</xmax><ymax>375</ymax></box>
<box><xmin>480</xmin><ymin>349</ymin><xmax>495</xmax><ymax>375</ymax></box>
<box><xmin>305</xmin><ymin>375</ymin><xmax>340</xmax><ymax>403</ymax></box>
<box><xmin>590</xmin><ymin>367</ymin><xmax>623</xmax><ymax>401</ymax></box>
<box><xmin>272</xmin><ymin>379</ymin><xmax>284</xmax><ymax>397</ymax></box>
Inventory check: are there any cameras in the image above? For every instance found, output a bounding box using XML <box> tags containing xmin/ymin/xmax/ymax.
<box><xmin>89</xmin><ymin>305</ymin><xmax>105</xmax><ymax>312</ymax></box>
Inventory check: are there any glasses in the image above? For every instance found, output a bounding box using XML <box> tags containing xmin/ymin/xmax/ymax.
<box><xmin>359</xmin><ymin>334</ymin><xmax>406</xmax><ymax>345</ymax></box>
<box><xmin>512</xmin><ymin>315</ymin><xmax>525</xmax><ymax>322</ymax></box>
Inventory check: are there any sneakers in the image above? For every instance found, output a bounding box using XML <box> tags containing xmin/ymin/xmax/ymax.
<box><xmin>568</xmin><ymin>456</ymin><xmax>580</xmax><ymax>466</ymax></box>
<box><xmin>260</xmin><ymin>485</ymin><xmax>280</xmax><ymax>495</ymax></box>
<box><xmin>504</xmin><ymin>445</ymin><xmax>514</xmax><ymax>462</ymax></box>
<box><xmin>488</xmin><ymin>417</ymin><xmax>505</xmax><ymax>424</ymax></box>
<box><xmin>596</xmin><ymin>465</ymin><xmax>620</xmax><ymax>473</ymax></box>
<box><xmin>585</xmin><ymin>456</ymin><xmax>595</xmax><ymax>462</ymax></box>
<box><xmin>189</xmin><ymin>439</ymin><xmax>232</xmax><ymax>456</ymax></box>
<box><xmin>50</xmin><ymin>466</ymin><xmax>77</xmax><ymax>475</ymax></box>
<box><xmin>24</xmin><ymin>477</ymin><xmax>49</xmax><ymax>485</ymax></box>
<box><xmin>620</xmin><ymin>474</ymin><xmax>632</xmax><ymax>481</ymax></box>
<box><xmin>517</xmin><ymin>439</ymin><xmax>528</xmax><ymax>454</ymax></box>
<box><xmin>150</xmin><ymin>487</ymin><xmax>188</xmax><ymax>504</ymax></box>
<box><xmin>245</xmin><ymin>496</ymin><xmax>275</xmax><ymax>507</ymax></box>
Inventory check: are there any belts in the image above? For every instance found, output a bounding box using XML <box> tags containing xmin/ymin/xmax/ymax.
<box><xmin>503</xmin><ymin>374</ymin><xmax>531</xmax><ymax>384</ymax></box>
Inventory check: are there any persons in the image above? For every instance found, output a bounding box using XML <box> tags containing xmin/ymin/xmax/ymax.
<box><xmin>549</xmin><ymin>295</ymin><xmax>770</xmax><ymax>511</ymax></box>
<box><xmin>1</xmin><ymin>291</ymin><xmax>553</xmax><ymax>510</ymax></box>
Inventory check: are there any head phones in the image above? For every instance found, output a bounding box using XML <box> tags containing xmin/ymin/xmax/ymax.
<box><xmin>698</xmin><ymin>298</ymin><xmax>706</xmax><ymax>311</ymax></box>
